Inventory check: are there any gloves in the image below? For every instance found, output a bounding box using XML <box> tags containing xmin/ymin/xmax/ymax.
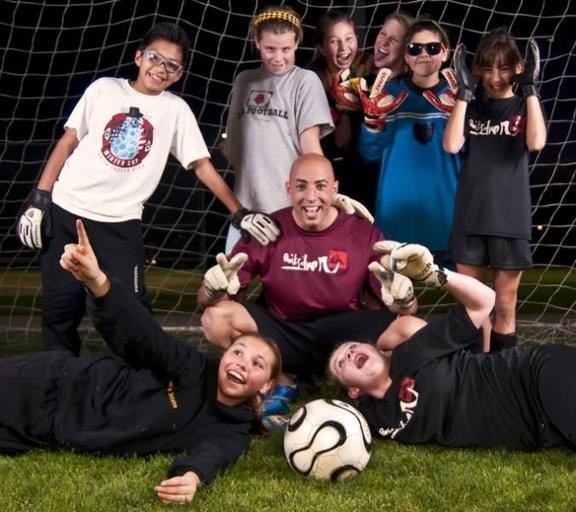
<box><xmin>357</xmin><ymin>67</ymin><xmax>408</xmax><ymax>132</ymax></box>
<box><xmin>204</xmin><ymin>249</ymin><xmax>249</xmax><ymax>299</ymax></box>
<box><xmin>368</xmin><ymin>255</ymin><xmax>416</xmax><ymax>310</ymax></box>
<box><xmin>422</xmin><ymin>66</ymin><xmax>461</xmax><ymax>117</ymax></box>
<box><xmin>451</xmin><ymin>44</ymin><xmax>479</xmax><ymax>103</ymax></box>
<box><xmin>16</xmin><ymin>190</ymin><xmax>54</xmax><ymax>253</ymax></box>
<box><xmin>515</xmin><ymin>37</ymin><xmax>541</xmax><ymax>96</ymax></box>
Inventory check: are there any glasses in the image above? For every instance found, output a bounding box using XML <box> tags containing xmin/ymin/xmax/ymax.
<box><xmin>146</xmin><ymin>48</ymin><xmax>183</xmax><ymax>73</ymax></box>
<box><xmin>408</xmin><ymin>42</ymin><xmax>443</xmax><ymax>56</ymax></box>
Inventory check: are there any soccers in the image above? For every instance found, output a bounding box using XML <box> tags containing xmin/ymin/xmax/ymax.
<box><xmin>283</xmin><ymin>398</ymin><xmax>373</xmax><ymax>483</ymax></box>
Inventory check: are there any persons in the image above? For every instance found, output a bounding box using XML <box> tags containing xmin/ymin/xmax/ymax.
<box><xmin>1</xmin><ymin>219</ymin><xmax>283</xmax><ymax>504</ymax></box>
<box><xmin>308</xmin><ymin>10</ymin><xmax>363</xmax><ymax>199</ymax></box>
<box><xmin>442</xmin><ymin>29</ymin><xmax>548</xmax><ymax>353</ymax></box>
<box><xmin>352</xmin><ymin>19</ymin><xmax>464</xmax><ymax>274</ymax></box>
<box><xmin>16</xmin><ymin>24</ymin><xmax>280</xmax><ymax>366</ymax></box>
<box><xmin>330</xmin><ymin>14</ymin><xmax>415</xmax><ymax>216</ymax></box>
<box><xmin>221</xmin><ymin>6</ymin><xmax>338</xmax><ymax>256</ymax></box>
<box><xmin>325</xmin><ymin>239</ymin><xmax>576</xmax><ymax>454</ymax></box>
<box><xmin>196</xmin><ymin>152</ymin><xmax>430</xmax><ymax>430</ymax></box>
